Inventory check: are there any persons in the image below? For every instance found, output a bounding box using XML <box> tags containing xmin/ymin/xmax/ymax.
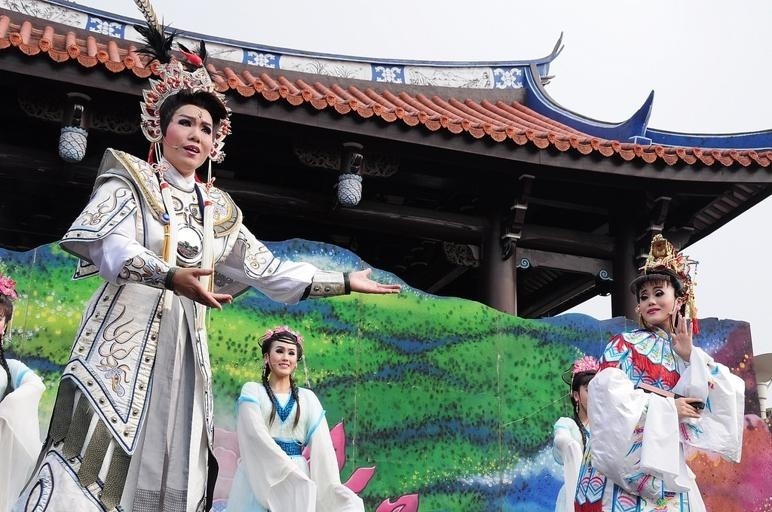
<box><xmin>19</xmin><ymin>92</ymin><xmax>405</xmax><ymax>512</ymax></box>
<box><xmin>1</xmin><ymin>272</ymin><xmax>46</xmax><ymax>512</ymax></box>
<box><xmin>573</xmin><ymin>266</ymin><xmax>748</xmax><ymax>512</ymax></box>
<box><xmin>546</xmin><ymin>354</ymin><xmax>609</xmax><ymax>512</ymax></box>
<box><xmin>225</xmin><ymin>325</ymin><xmax>364</xmax><ymax>511</ymax></box>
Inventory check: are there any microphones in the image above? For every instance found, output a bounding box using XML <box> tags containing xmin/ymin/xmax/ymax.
<box><xmin>668</xmin><ymin>301</ymin><xmax>678</xmax><ymax>315</ymax></box>
<box><xmin>163</xmin><ymin>137</ymin><xmax>178</xmax><ymax>151</ymax></box>
